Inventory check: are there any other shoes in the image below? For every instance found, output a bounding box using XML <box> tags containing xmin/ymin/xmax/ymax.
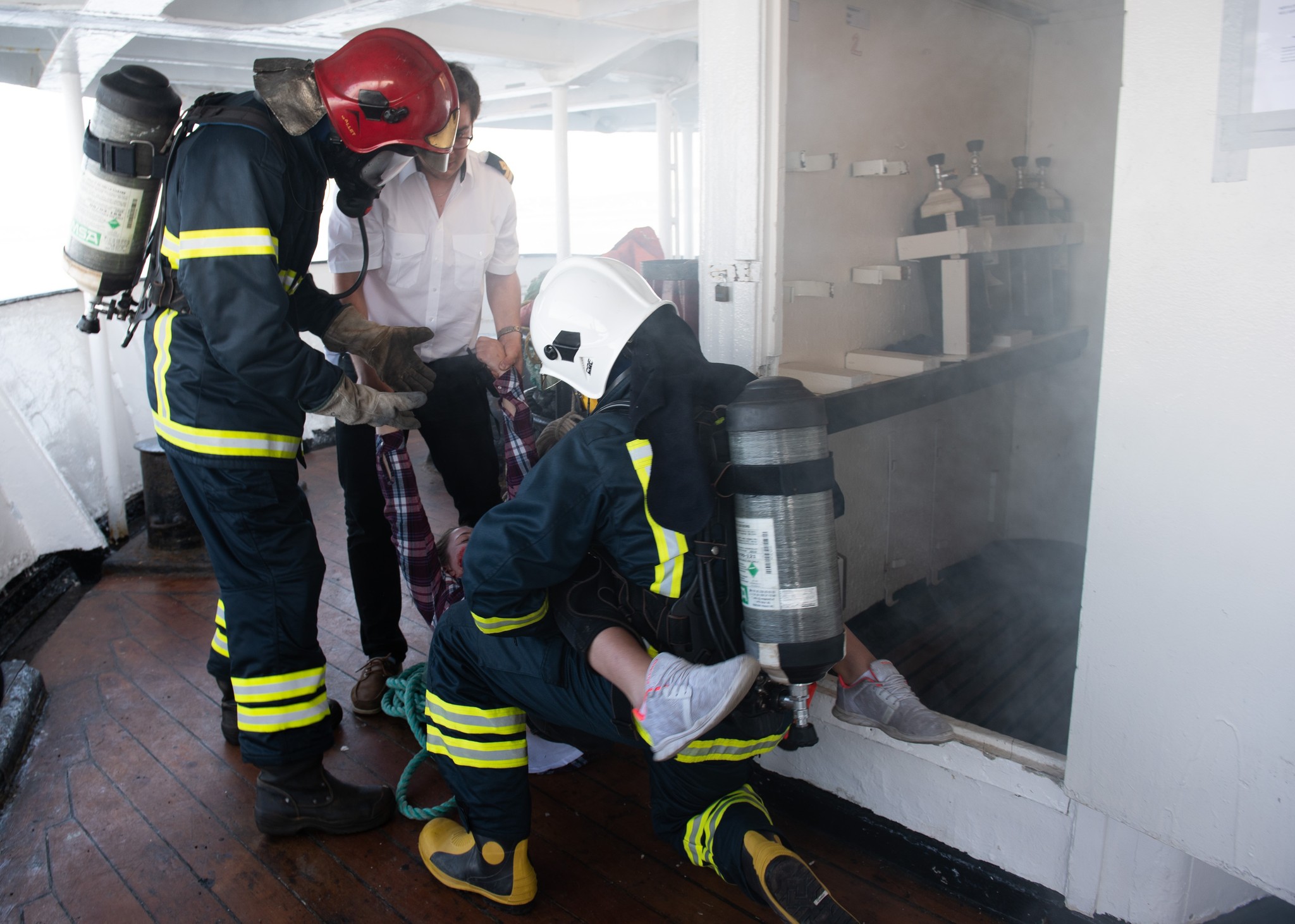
<box><xmin>255</xmin><ymin>765</ymin><xmax>395</xmax><ymax>834</ymax></box>
<box><xmin>220</xmin><ymin>695</ymin><xmax>345</xmax><ymax>743</ymax></box>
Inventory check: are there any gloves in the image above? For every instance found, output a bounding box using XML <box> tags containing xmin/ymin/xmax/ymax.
<box><xmin>325</xmin><ymin>311</ymin><xmax>436</xmax><ymax>396</ymax></box>
<box><xmin>296</xmin><ymin>373</ymin><xmax>426</xmax><ymax>429</ymax></box>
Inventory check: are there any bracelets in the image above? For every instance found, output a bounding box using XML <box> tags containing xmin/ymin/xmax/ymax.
<box><xmin>496</xmin><ymin>325</ymin><xmax>523</xmax><ymax>340</ymax></box>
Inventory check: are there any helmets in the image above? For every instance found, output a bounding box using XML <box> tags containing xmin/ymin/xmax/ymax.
<box><xmin>314</xmin><ymin>26</ymin><xmax>461</xmax><ymax>154</ymax></box>
<box><xmin>530</xmin><ymin>255</ymin><xmax>682</xmax><ymax>398</ymax></box>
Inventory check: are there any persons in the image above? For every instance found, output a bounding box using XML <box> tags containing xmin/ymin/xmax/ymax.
<box><xmin>143</xmin><ymin>26</ymin><xmax>461</xmax><ymax>838</ymax></box>
<box><xmin>327</xmin><ymin>60</ymin><xmax>522</xmax><ymax>715</ymax></box>
<box><xmin>375</xmin><ymin>254</ymin><xmax>956</xmax><ymax>923</ymax></box>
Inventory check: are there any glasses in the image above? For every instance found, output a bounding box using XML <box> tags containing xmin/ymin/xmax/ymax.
<box><xmin>454</xmin><ymin>121</ymin><xmax>476</xmax><ymax>150</ymax></box>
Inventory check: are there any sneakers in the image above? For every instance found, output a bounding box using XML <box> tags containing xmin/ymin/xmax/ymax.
<box><xmin>351</xmin><ymin>656</ymin><xmax>402</xmax><ymax>712</ymax></box>
<box><xmin>631</xmin><ymin>651</ymin><xmax>761</xmax><ymax>762</ymax></box>
<box><xmin>833</xmin><ymin>658</ymin><xmax>957</xmax><ymax>744</ymax></box>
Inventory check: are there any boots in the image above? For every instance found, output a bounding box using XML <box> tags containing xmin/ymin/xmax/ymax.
<box><xmin>743</xmin><ymin>832</ymin><xmax>862</xmax><ymax>924</ymax></box>
<box><xmin>417</xmin><ymin>817</ymin><xmax>538</xmax><ymax>905</ymax></box>
